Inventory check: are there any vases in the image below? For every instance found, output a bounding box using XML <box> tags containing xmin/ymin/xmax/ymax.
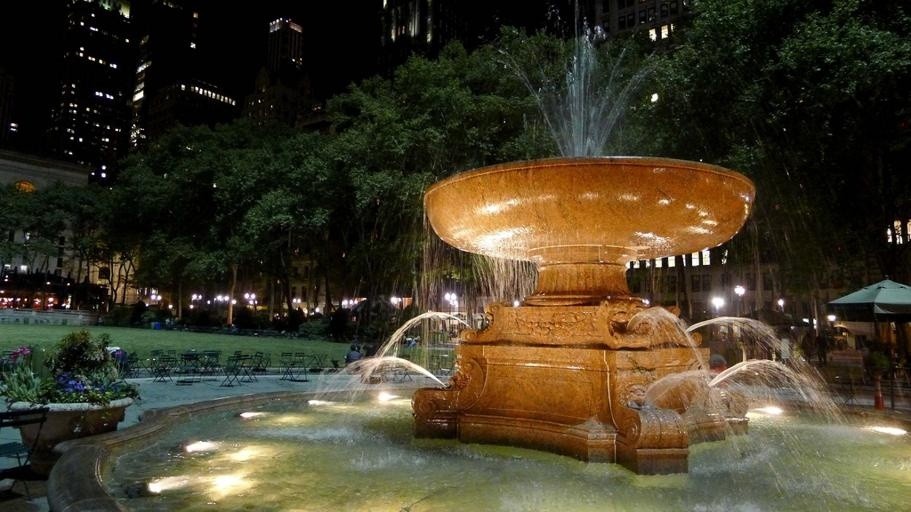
<box><xmin>6</xmin><ymin>397</ymin><xmax>134</xmax><ymax>478</ymax></box>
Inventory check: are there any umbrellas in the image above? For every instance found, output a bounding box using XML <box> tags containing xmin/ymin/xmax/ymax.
<box><xmin>743</xmin><ymin>308</ymin><xmax>810</xmax><ymax>327</ymax></box>
<box><xmin>827</xmin><ymin>278</ymin><xmax>911</xmax><ymax>324</ymax></box>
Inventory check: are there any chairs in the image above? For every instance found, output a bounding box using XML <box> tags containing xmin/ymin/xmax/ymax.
<box><xmin>125</xmin><ymin>349</ymin><xmax>454</xmax><ymax>388</ymax></box>
<box><xmin>834</xmin><ymin>347</ymin><xmax>910</xmax><ymax>409</ymax></box>
<box><xmin>0</xmin><ymin>407</ymin><xmax>49</xmax><ymax>501</ymax></box>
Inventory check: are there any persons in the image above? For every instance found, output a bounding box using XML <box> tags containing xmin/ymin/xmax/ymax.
<box><xmin>802</xmin><ymin>331</ymin><xmax>814</xmax><ymax>363</ymax></box>
<box><xmin>345</xmin><ymin>343</ymin><xmax>380</xmax><ymax>369</ymax></box>
<box><xmin>815</xmin><ymin>332</ymin><xmax>828</xmax><ymax>361</ymax></box>
<box><xmin>404</xmin><ymin>335</ymin><xmax>422</xmax><ymax>349</ymax></box>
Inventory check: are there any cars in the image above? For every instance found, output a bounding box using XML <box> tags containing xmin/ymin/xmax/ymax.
<box><xmin>830</xmin><ymin>324</ymin><xmax>852</xmax><ymax>348</ymax></box>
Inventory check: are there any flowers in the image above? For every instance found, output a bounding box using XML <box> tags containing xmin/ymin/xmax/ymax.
<box><xmin>0</xmin><ymin>328</ymin><xmax>141</xmax><ymax>424</ymax></box>
